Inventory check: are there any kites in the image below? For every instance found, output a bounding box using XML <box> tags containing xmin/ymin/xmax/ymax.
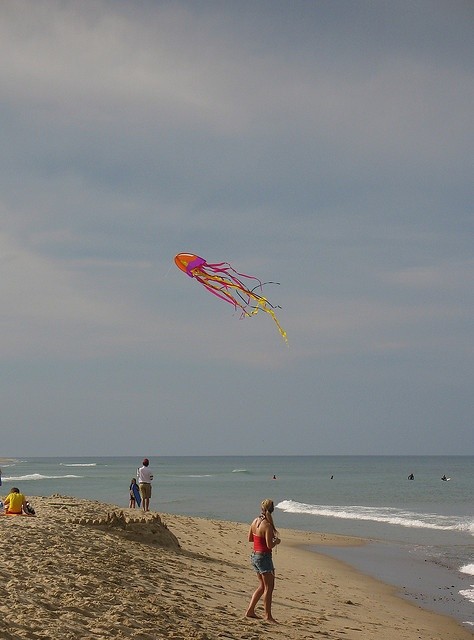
<box><xmin>173</xmin><ymin>250</ymin><xmax>288</xmax><ymax>343</ymax></box>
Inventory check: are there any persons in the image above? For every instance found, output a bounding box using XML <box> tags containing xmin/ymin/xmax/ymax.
<box><xmin>130</xmin><ymin>478</ymin><xmax>139</xmax><ymax>508</ymax></box>
<box><xmin>271</xmin><ymin>475</ymin><xmax>279</xmax><ymax>480</ymax></box>
<box><xmin>136</xmin><ymin>459</ymin><xmax>153</xmax><ymax>511</ymax></box>
<box><xmin>408</xmin><ymin>473</ymin><xmax>415</xmax><ymax>479</ymax></box>
<box><xmin>442</xmin><ymin>475</ymin><xmax>449</xmax><ymax>480</ymax></box>
<box><xmin>330</xmin><ymin>475</ymin><xmax>334</xmax><ymax>480</ymax></box>
<box><xmin>246</xmin><ymin>499</ymin><xmax>281</xmax><ymax>624</ymax></box>
<box><xmin>2</xmin><ymin>487</ymin><xmax>30</xmax><ymax>514</ymax></box>
